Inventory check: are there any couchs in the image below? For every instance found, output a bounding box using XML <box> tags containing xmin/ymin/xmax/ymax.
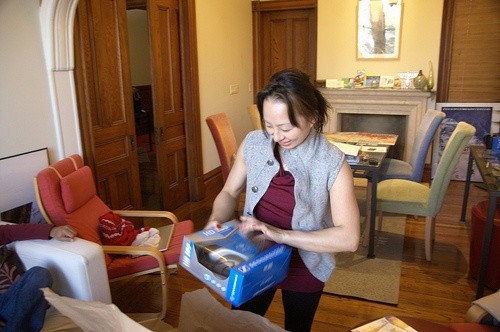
<box><xmin>0</xmin><ymin>218</ymin><xmax>110</xmax><ymax>332</ymax></box>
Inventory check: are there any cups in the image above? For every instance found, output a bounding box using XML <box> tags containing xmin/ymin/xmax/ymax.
<box><xmin>492</xmin><ymin>137</ymin><xmax>500</xmax><ymax>152</ymax></box>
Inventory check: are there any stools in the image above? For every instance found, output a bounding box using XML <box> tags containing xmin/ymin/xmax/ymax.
<box><xmin>469</xmin><ymin>198</ymin><xmax>500</xmax><ymax>289</ymax></box>
<box><xmin>465</xmin><ymin>290</ymin><xmax>500</xmax><ymax>332</ymax></box>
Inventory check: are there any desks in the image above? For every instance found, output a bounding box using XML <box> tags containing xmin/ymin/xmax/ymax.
<box><xmin>323</xmin><ymin>132</ymin><xmax>396</xmax><ymax>266</ymax></box>
<box><xmin>460</xmin><ymin>145</ymin><xmax>500</xmax><ymax>300</ymax></box>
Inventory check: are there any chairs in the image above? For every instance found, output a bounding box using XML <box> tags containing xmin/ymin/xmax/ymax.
<box><xmin>362</xmin><ymin>109</ymin><xmax>475</xmax><ymax>262</ymax></box>
<box><xmin>206</xmin><ymin>111</ymin><xmax>247</xmax><ymax>211</ymax></box>
<box><xmin>35</xmin><ymin>154</ymin><xmax>195</xmax><ymax>319</ymax></box>
<box><xmin>247</xmin><ymin>105</ymin><xmax>262</xmax><ymax>130</ymax></box>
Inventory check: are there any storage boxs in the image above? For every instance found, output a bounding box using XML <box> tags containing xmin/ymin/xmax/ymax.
<box><xmin>180</xmin><ymin>218</ymin><xmax>293</xmax><ymax>305</ymax></box>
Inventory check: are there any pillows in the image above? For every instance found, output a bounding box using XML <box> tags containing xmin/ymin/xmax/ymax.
<box><xmin>62</xmin><ymin>166</ymin><xmax>96</xmax><ymax>213</ymax></box>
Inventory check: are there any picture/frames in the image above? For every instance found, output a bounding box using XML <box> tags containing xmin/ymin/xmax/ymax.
<box><xmin>356</xmin><ymin>0</ymin><xmax>404</xmax><ymax>60</ymax></box>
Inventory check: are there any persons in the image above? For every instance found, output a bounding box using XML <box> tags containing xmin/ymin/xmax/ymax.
<box><xmin>202</xmin><ymin>70</ymin><xmax>360</xmax><ymax>332</ymax></box>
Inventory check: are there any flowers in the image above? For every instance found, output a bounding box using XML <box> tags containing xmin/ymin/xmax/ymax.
<box><xmin>432</xmin><ymin>102</ymin><xmax>500</xmax><ymax>181</ymax></box>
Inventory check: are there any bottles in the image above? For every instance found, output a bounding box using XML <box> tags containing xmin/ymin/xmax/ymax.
<box><xmin>413</xmin><ymin>70</ymin><xmax>427</xmax><ymax>89</ymax></box>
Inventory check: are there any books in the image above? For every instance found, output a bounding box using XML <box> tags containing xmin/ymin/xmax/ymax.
<box><xmin>334</xmin><ymin>142</ymin><xmax>360</xmax><ymax>165</ymax></box>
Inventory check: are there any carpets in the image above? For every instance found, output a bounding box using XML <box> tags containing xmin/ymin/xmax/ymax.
<box><xmin>322</xmin><ymin>188</ymin><xmax>407</xmax><ymax>307</ymax></box>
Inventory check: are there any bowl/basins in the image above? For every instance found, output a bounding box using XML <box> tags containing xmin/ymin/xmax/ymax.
<box><xmin>483</xmin><ymin>134</ymin><xmax>500</xmax><ymax>149</ymax></box>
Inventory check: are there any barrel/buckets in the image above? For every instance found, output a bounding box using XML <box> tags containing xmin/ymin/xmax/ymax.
<box><xmin>470</xmin><ymin>199</ymin><xmax>500</xmax><ymax>289</ymax></box>
